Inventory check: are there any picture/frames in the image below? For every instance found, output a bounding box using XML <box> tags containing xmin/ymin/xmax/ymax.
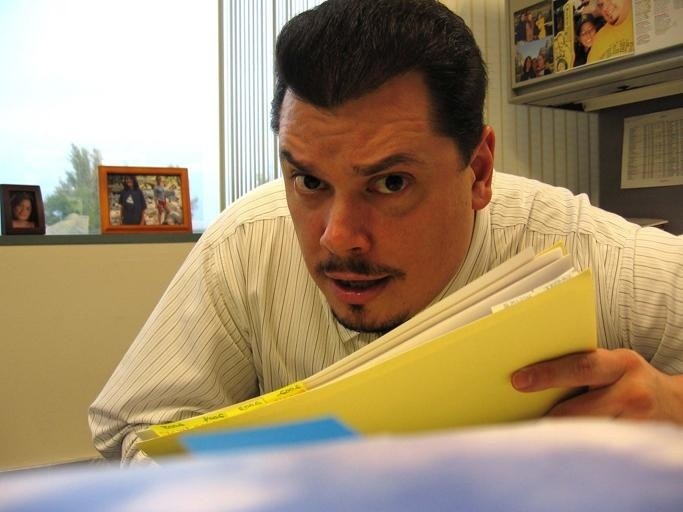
<box><xmin>0</xmin><ymin>182</ymin><xmax>46</xmax><ymax>236</ymax></box>
<box><xmin>94</xmin><ymin>163</ymin><xmax>194</xmax><ymax>234</ymax></box>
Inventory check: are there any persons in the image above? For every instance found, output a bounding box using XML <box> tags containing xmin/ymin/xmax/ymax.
<box><xmin>87</xmin><ymin>0</ymin><xmax>683</xmax><ymax>466</ymax></box>
<box><xmin>154</xmin><ymin>176</ymin><xmax>170</xmax><ymax>225</ymax></box>
<box><xmin>573</xmin><ymin>17</ymin><xmax>600</xmax><ymax>67</ymax></box>
<box><xmin>10</xmin><ymin>192</ymin><xmax>36</xmax><ymax>228</ymax></box>
<box><xmin>587</xmin><ymin>0</ymin><xmax>634</xmax><ymax>64</ymax></box>
<box><xmin>516</xmin><ymin>11</ymin><xmax>551</xmax><ymax>82</ymax></box>
<box><xmin>119</xmin><ymin>175</ymin><xmax>147</xmax><ymax>225</ymax></box>
